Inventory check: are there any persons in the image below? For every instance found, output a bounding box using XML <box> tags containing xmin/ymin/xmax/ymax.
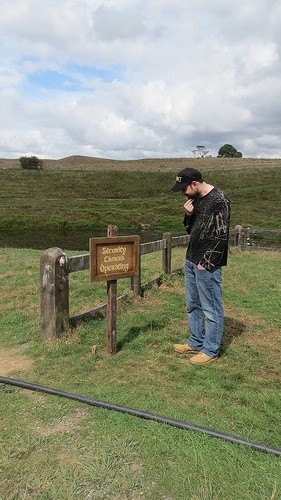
<box><xmin>170</xmin><ymin>169</ymin><xmax>230</xmax><ymax>364</ymax></box>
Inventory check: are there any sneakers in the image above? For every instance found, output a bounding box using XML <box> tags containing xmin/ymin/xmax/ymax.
<box><xmin>189</xmin><ymin>351</ymin><xmax>218</xmax><ymax>364</ymax></box>
<box><xmin>173</xmin><ymin>343</ymin><xmax>200</xmax><ymax>354</ymax></box>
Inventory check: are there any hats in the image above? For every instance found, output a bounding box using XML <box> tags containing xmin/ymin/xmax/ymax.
<box><xmin>171</xmin><ymin>167</ymin><xmax>202</xmax><ymax>192</ymax></box>
